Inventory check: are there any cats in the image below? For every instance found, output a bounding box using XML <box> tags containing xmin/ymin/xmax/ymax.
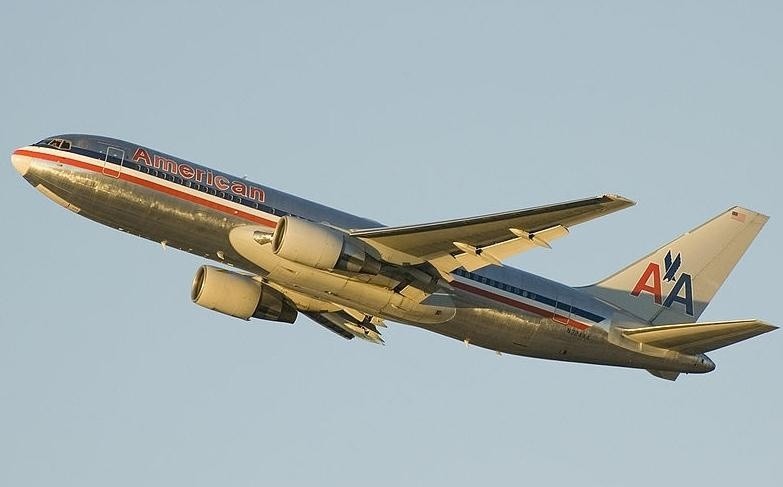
<box><xmin>10</xmin><ymin>133</ymin><xmax>783</xmax><ymax>382</ymax></box>
